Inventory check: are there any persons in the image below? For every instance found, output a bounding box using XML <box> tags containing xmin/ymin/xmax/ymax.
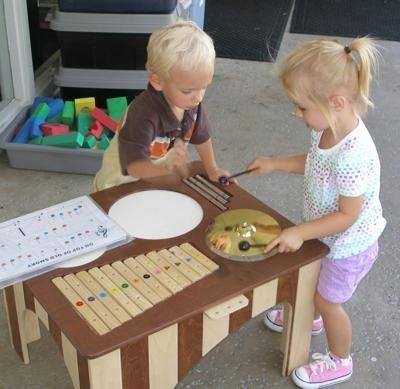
<box><xmin>91</xmin><ymin>19</ymin><xmax>238</xmax><ymax>196</ymax></box>
<box><xmin>244</xmin><ymin>34</ymin><xmax>390</xmax><ymax>389</ymax></box>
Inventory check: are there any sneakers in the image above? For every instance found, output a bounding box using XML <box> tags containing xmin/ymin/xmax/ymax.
<box><xmin>263</xmin><ymin>308</ymin><xmax>325</xmax><ymax>335</ymax></box>
<box><xmin>290</xmin><ymin>350</ymin><xmax>357</xmax><ymax>389</ymax></box>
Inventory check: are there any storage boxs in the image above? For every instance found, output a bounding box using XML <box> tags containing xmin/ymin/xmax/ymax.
<box><xmin>0</xmin><ymin>0</ymin><xmax>204</xmax><ymax>176</ymax></box>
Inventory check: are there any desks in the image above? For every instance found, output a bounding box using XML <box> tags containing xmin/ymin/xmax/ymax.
<box><xmin>1</xmin><ymin>159</ymin><xmax>330</xmax><ymax>389</ymax></box>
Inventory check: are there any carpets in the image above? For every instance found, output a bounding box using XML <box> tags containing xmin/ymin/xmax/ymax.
<box><xmin>288</xmin><ymin>0</ymin><xmax>399</xmax><ymax>42</ymax></box>
<box><xmin>204</xmin><ymin>0</ymin><xmax>293</xmax><ymax>62</ymax></box>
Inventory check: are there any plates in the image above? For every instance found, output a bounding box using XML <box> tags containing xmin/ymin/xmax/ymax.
<box><xmin>108</xmin><ymin>190</ymin><xmax>204</xmax><ymax>240</ymax></box>
